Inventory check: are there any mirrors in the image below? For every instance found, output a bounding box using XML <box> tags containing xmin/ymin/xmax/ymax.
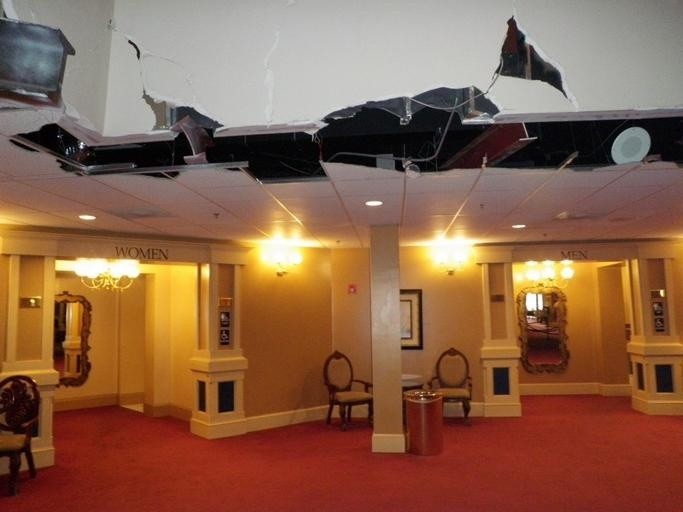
<box><xmin>54</xmin><ymin>291</ymin><xmax>92</xmax><ymax>388</ymax></box>
<box><xmin>515</xmin><ymin>283</ymin><xmax>570</xmax><ymax>374</ymax></box>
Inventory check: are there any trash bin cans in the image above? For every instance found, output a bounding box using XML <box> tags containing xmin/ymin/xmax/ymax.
<box><xmin>404</xmin><ymin>388</ymin><xmax>443</xmax><ymax>456</ymax></box>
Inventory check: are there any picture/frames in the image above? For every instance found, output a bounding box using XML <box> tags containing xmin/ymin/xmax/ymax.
<box><xmin>400</xmin><ymin>289</ymin><xmax>423</xmax><ymax>350</ymax></box>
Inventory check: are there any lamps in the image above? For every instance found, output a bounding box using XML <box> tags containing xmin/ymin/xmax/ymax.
<box><xmin>260</xmin><ymin>248</ymin><xmax>303</xmax><ymax>276</ymax></box>
<box><xmin>525</xmin><ymin>261</ymin><xmax>574</xmax><ymax>288</ymax></box>
<box><xmin>429</xmin><ymin>238</ymin><xmax>470</xmax><ymax>276</ymax></box>
<box><xmin>73</xmin><ymin>257</ymin><xmax>141</xmax><ymax>293</ymax></box>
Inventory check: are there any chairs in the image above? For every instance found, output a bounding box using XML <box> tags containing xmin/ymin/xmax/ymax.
<box><xmin>323</xmin><ymin>351</ymin><xmax>374</xmax><ymax>431</ymax></box>
<box><xmin>427</xmin><ymin>347</ymin><xmax>473</xmax><ymax>426</ymax></box>
<box><xmin>0</xmin><ymin>375</ymin><xmax>41</xmax><ymax>495</ymax></box>
